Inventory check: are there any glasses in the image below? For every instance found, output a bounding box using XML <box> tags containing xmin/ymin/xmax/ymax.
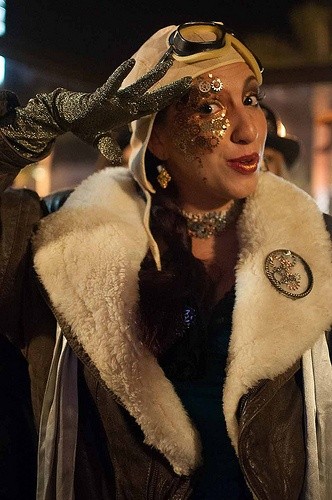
<box><xmin>156</xmin><ymin>21</ymin><xmax>264</xmax><ymax>86</ymax></box>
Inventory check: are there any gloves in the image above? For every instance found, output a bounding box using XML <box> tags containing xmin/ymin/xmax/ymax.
<box><xmin>0</xmin><ymin>58</ymin><xmax>194</xmax><ymax>165</ymax></box>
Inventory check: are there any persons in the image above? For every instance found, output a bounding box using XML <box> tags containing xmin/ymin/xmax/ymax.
<box><xmin>0</xmin><ymin>19</ymin><xmax>332</xmax><ymax>500</ymax></box>
<box><xmin>253</xmin><ymin>104</ymin><xmax>331</xmax><ymax>238</ymax></box>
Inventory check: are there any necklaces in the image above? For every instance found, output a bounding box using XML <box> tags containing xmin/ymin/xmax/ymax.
<box><xmin>182</xmin><ymin>198</ymin><xmax>241</xmax><ymax>238</ymax></box>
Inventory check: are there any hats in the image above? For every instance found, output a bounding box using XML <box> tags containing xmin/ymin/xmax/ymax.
<box><xmin>116</xmin><ymin>25</ymin><xmax>245</xmax><ymax>271</ymax></box>
<box><xmin>259</xmin><ymin>102</ymin><xmax>301</xmax><ymax>171</ymax></box>
